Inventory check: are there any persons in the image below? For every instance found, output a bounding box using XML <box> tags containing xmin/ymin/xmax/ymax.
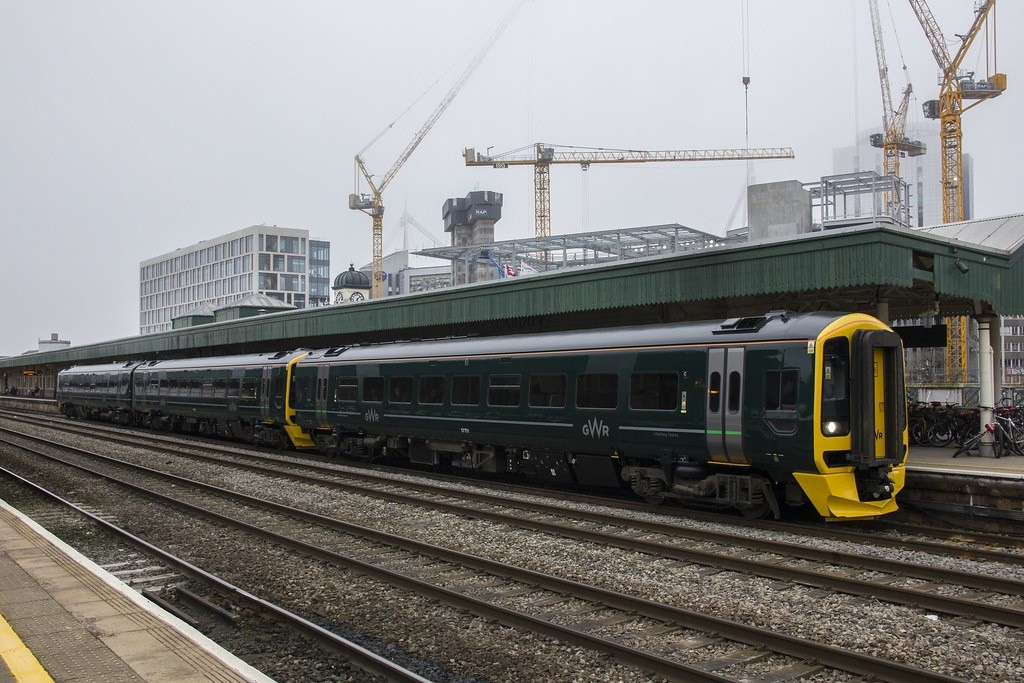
<box><xmin>9</xmin><ymin>386</ymin><xmax>15</xmax><ymax>394</ymax></box>
<box><xmin>424</xmin><ymin>388</ymin><xmax>440</xmax><ymax>403</ymax></box>
<box><xmin>830</xmin><ymin>356</ymin><xmax>848</xmax><ymax>422</ymax></box>
<box><xmin>369</xmin><ymin>388</ymin><xmax>378</xmax><ymax>401</ymax></box>
<box><xmin>781</xmin><ymin>381</ymin><xmax>795</xmax><ymax>404</ymax></box>
<box><xmin>32</xmin><ymin>386</ymin><xmax>40</xmax><ymax>397</ymax></box>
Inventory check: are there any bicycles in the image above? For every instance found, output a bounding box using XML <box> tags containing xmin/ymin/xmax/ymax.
<box><xmin>906</xmin><ymin>399</ymin><xmax>1024</xmax><ymax>459</ymax></box>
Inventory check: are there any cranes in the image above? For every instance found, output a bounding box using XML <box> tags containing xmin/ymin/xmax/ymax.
<box><xmin>347</xmin><ymin>2</ymin><xmax>523</xmax><ymax>302</ymax></box>
<box><xmin>460</xmin><ymin>145</ymin><xmax>797</xmax><ymax>267</ymax></box>
<box><xmin>865</xmin><ymin>2</ymin><xmax>1008</xmax><ymax>384</ymax></box>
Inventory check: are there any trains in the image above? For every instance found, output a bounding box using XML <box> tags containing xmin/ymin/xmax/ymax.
<box><xmin>55</xmin><ymin>312</ymin><xmax>910</xmax><ymax>525</ymax></box>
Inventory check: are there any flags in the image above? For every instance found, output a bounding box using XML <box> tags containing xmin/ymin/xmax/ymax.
<box><xmin>521</xmin><ymin>259</ymin><xmax>538</xmax><ymax>276</ymax></box>
<box><xmin>504</xmin><ymin>264</ymin><xmax>517</xmax><ymax>278</ymax></box>
